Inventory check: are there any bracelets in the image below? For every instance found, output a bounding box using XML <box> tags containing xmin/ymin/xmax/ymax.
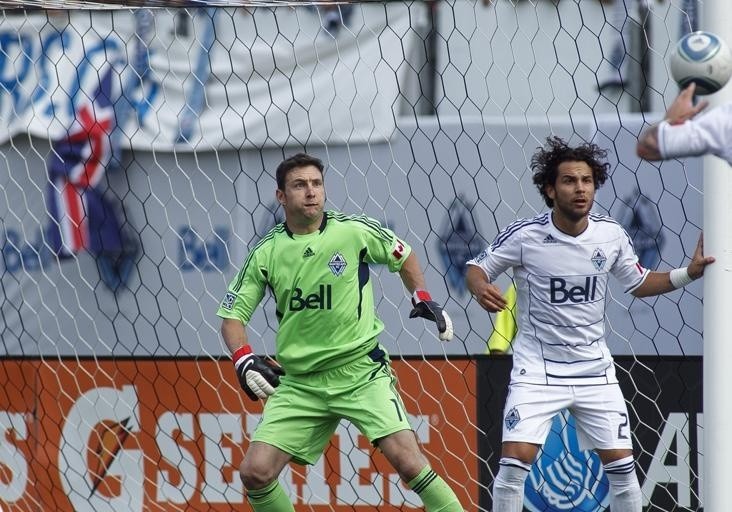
<box><xmin>669</xmin><ymin>267</ymin><xmax>694</xmax><ymax>289</ymax></box>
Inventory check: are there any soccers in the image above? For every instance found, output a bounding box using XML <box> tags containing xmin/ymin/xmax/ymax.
<box><xmin>668</xmin><ymin>31</ymin><xmax>732</xmax><ymax>95</ymax></box>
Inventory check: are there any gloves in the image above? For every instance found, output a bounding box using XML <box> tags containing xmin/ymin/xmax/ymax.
<box><xmin>409</xmin><ymin>300</ymin><xmax>454</xmax><ymax>341</ymax></box>
<box><xmin>233</xmin><ymin>352</ymin><xmax>285</xmax><ymax>401</ymax></box>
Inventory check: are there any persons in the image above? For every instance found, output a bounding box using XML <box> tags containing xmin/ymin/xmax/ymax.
<box><xmin>216</xmin><ymin>153</ymin><xmax>464</xmax><ymax>512</ymax></box>
<box><xmin>486</xmin><ymin>281</ymin><xmax>517</xmax><ymax>355</ymax></box>
<box><xmin>465</xmin><ymin>135</ymin><xmax>716</xmax><ymax>512</ymax></box>
<box><xmin>637</xmin><ymin>81</ymin><xmax>731</xmax><ymax>167</ymax></box>
